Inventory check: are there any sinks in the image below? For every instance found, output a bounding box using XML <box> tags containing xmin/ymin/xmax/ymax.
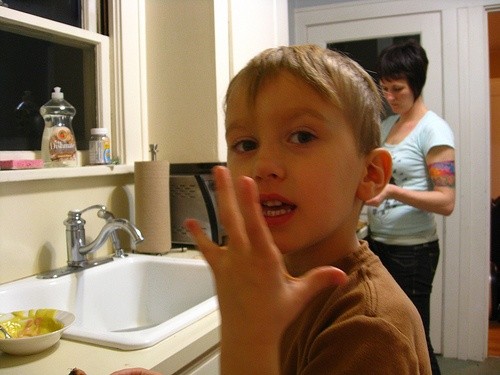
<box><xmin>0</xmin><ymin>250</ymin><xmax>218</xmax><ymax>347</ymax></box>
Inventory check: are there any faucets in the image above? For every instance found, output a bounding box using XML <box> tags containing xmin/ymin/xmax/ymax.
<box><xmin>58</xmin><ymin>212</ymin><xmax>141</xmax><ymax>261</ymax></box>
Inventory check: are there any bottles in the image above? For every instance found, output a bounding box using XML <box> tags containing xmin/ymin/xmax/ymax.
<box><xmin>40</xmin><ymin>87</ymin><xmax>80</xmax><ymax>167</ymax></box>
<box><xmin>88</xmin><ymin>126</ymin><xmax>112</xmax><ymax>165</ymax></box>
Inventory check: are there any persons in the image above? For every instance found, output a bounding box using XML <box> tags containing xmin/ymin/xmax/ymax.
<box><xmin>354</xmin><ymin>35</ymin><xmax>457</xmax><ymax>374</ymax></box>
<box><xmin>182</xmin><ymin>43</ymin><xmax>434</xmax><ymax>375</ymax></box>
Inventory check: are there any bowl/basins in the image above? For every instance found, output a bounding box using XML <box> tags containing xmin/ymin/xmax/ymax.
<box><xmin>0</xmin><ymin>307</ymin><xmax>75</xmax><ymax>355</ymax></box>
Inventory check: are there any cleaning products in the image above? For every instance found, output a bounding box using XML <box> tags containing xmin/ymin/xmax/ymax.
<box><xmin>40</xmin><ymin>86</ymin><xmax>77</xmax><ymax>166</ymax></box>
